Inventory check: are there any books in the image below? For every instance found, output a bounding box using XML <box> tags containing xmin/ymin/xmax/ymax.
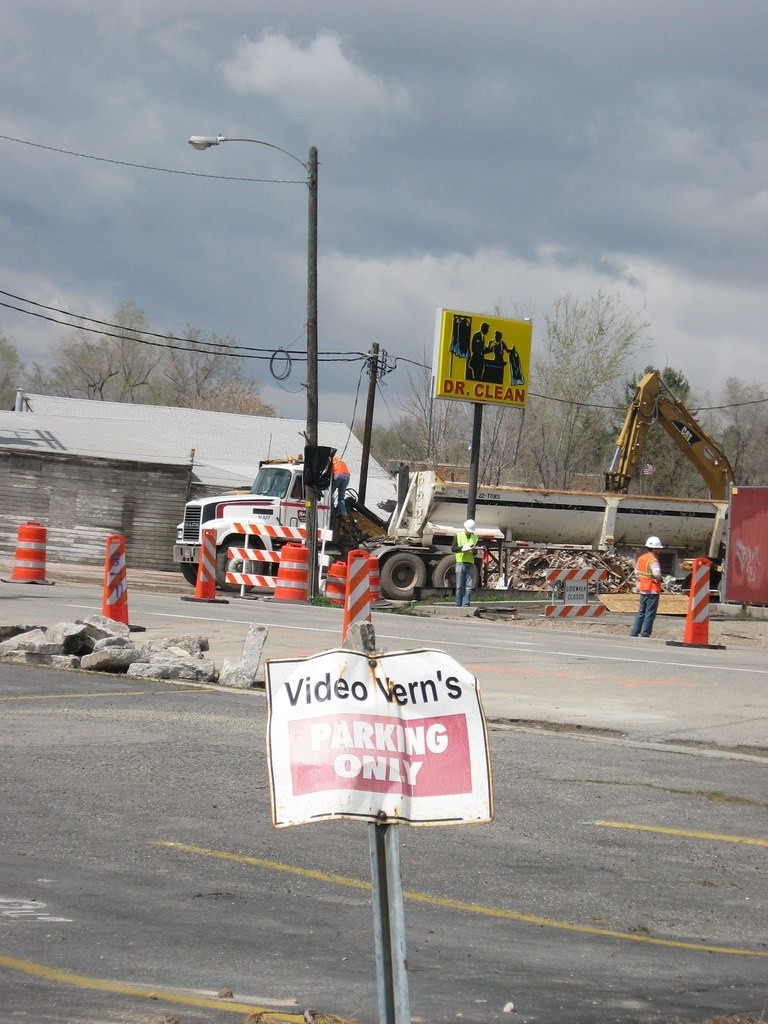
<box><xmin>463</xmin><ymin>545</ymin><xmax>489</xmax><ymax>565</ymax></box>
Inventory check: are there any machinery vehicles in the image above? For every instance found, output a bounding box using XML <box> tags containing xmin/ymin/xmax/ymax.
<box><xmin>603</xmin><ymin>367</ymin><xmax>739</xmax><ymax>601</ymax></box>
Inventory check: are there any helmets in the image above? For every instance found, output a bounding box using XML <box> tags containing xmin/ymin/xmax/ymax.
<box><xmin>464</xmin><ymin>519</ymin><xmax>475</xmax><ymax>533</ymax></box>
<box><xmin>645</xmin><ymin>536</ymin><xmax>664</xmax><ymax>548</ymax></box>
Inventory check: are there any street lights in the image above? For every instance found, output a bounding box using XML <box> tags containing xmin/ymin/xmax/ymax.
<box><xmin>186</xmin><ymin>132</ymin><xmax>324</xmax><ymax>610</ymax></box>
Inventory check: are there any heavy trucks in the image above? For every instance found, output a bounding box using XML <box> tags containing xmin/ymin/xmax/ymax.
<box><xmin>172</xmin><ymin>458</ymin><xmax>504</xmax><ymax>600</ymax></box>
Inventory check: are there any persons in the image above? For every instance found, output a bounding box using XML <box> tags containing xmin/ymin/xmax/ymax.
<box><xmin>451</xmin><ymin>519</ymin><xmax>478</xmax><ymax>607</ymax></box>
<box><xmin>631</xmin><ymin>536</ymin><xmax>665</xmax><ymax>638</ymax></box>
<box><xmin>331</xmin><ymin>453</ymin><xmax>350</xmax><ymax>520</ymax></box>
